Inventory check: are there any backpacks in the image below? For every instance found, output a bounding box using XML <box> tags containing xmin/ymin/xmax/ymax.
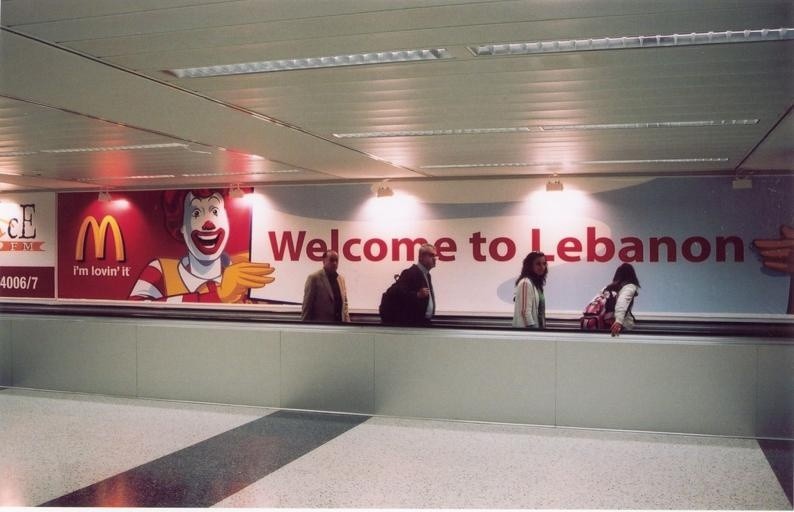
<box><xmin>577</xmin><ymin>280</ymin><xmax>623</xmax><ymax>333</ymax></box>
<box><xmin>378</xmin><ymin>269</ymin><xmax>410</xmax><ymax>325</ymax></box>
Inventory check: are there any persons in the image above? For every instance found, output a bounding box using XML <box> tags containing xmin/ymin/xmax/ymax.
<box><xmin>300</xmin><ymin>249</ymin><xmax>352</xmax><ymax>322</ymax></box>
<box><xmin>511</xmin><ymin>251</ymin><xmax>549</xmax><ymax>328</ymax></box>
<box><xmin>127</xmin><ymin>188</ymin><xmax>276</xmax><ymax>304</ymax></box>
<box><xmin>380</xmin><ymin>243</ymin><xmax>438</xmax><ymax>329</ymax></box>
<box><xmin>752</xmin><ymin>224</ymin><xmax>794</xmax><ymax>315</ymax></box>
<box><xmin>602</xmin><ymin>262</ymin><xmax>642</xmax><ymax>337</ymax></box>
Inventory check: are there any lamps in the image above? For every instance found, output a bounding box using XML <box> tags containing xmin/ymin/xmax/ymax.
<box><xmin>228</xmin><ymin>185</ymin><xmax>246</xmax><ymax>198</ymax></box>
<box><xmin>375</xmin><ymin>185</ymin><xmax>393</xmax><ymax>199</ymax></box>
<box><xmin>97</xmin><ymin>189</ymin><xmax>113</xmax><ymax>203</ymax></box>
<box><xmin>545</xmin><ymin>178</ymin><xmax>564</xmax><ymax>191</ymax></box>
<box><xmin>731</xmin><ymin>177</ymin><xmax>754</xmax><ymax>189</ymax></box>
